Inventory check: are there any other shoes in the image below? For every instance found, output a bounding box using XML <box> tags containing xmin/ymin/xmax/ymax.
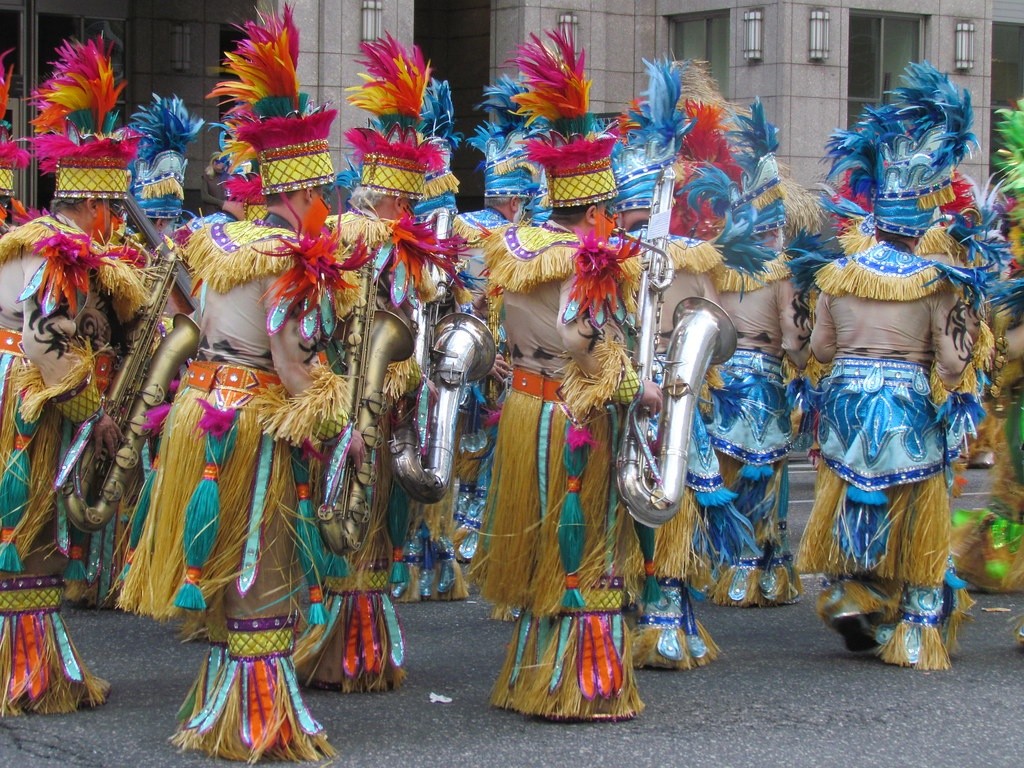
<box><xmin>834</xmin><ymin>615</ymin><xmax>880</xmax><ymax>652</ymax></box>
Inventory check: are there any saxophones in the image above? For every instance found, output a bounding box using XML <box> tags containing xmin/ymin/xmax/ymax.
<box><xmin>318</xmin><ymin>218</ymin><xmax>415</xmax><ymax>556</ymax></box>
<box><xmin>960</xmin><ymin>207</ymin><xmax>1010</xmax><ymax>419</ymax></box>
<box><xmin>60</xmin><ymin>230</ymin><xmax>204</xmax><ymax>534</ymax></box>
<box><xmin>389</xmin><ymin>207</ymin><xmax>496</xmax><ymax>505</ymax></box>
<box><xmin>610</xmin><ymin>164</ymin><xmax>741</xmax><ymax>527</ymax></box>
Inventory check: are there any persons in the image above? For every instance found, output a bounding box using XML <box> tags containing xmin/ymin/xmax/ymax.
<box><xmin>468</xmin><ymin>159</ymin><xmax>671</xmax><ymax>717</ymax></box>
<box><xmin>381</xmin><ymin>135</ymin><xmax>683</xmax><ymax>601</ymax></box>
<box><xmin>694</xmin><ymin>186</ymin><xmax>815</xmax><ymax>608</ymax></box>
<box><xmin>0</xmin><ymin>128</ymin><xmax>366</xmax><ymax>763</ymax></box>
<box><xmin>591</xmin><ymin>159</ymin><xmax>765</xmax><ymax>672</ymax></box>
<box><xmin>287</xmin><ymin>152</ymin><xmax>429</xmax><ymax>695</ymax></box>
<box><xmin>789</xmin><ymin>186</ymin><xmax>985</xmax><ymax>670</ymax></box>
<box><xmin>839</xmin><ymin>171</ymin><xmax>1023</xmax><ymax>647</ymax></box>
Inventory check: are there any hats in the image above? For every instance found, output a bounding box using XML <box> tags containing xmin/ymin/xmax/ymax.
<box><xmin>53</xmin><ymin>157</ymin><xmax>127</xmax><ymax>199</ymax></box>
<box><xmin>547</xmin><ymin>156</ymin><xmax>619</xmax><ymax>208</ymax></box>
<box><xmin>361</xmin><ymin>153</ymin><xmax>427</xmax><ymax>201</ymax></box>
<box><xmin>874</xmin><ymin>198</ymin><xmax>936</xmax><ymax>237</ymax></box>
<box><xmin>243</xmin><ymin>202</ymin><xmax>267</xmax><ymax>223</ymax></box>
<box><xmin>257</xmin><ymin>140</ymin><xmax>334</xmax><ymax>195</ymax></box>
<box><xmin>0</xmin><ymin>167</ymin><xmax>15</xmax><ymax>196</ymax></box>
<box><xmin>613</xmin><ymin>171</ymin><xmax>663</xmax><ymax>214</ymax></box>
<box><xmin>733</xmin><ymin>177</ymin><xmax>786</xmax><ymax>234</ymax></box>
<box><xmin>134</xmin><ymin>179</ymin><xmax>184</xmax><ymax>218</ymax></box>
<box><xmin>485</xmin><ymin>168</ymin><xmax>531</xmax><ymax>198</ymax></box>
